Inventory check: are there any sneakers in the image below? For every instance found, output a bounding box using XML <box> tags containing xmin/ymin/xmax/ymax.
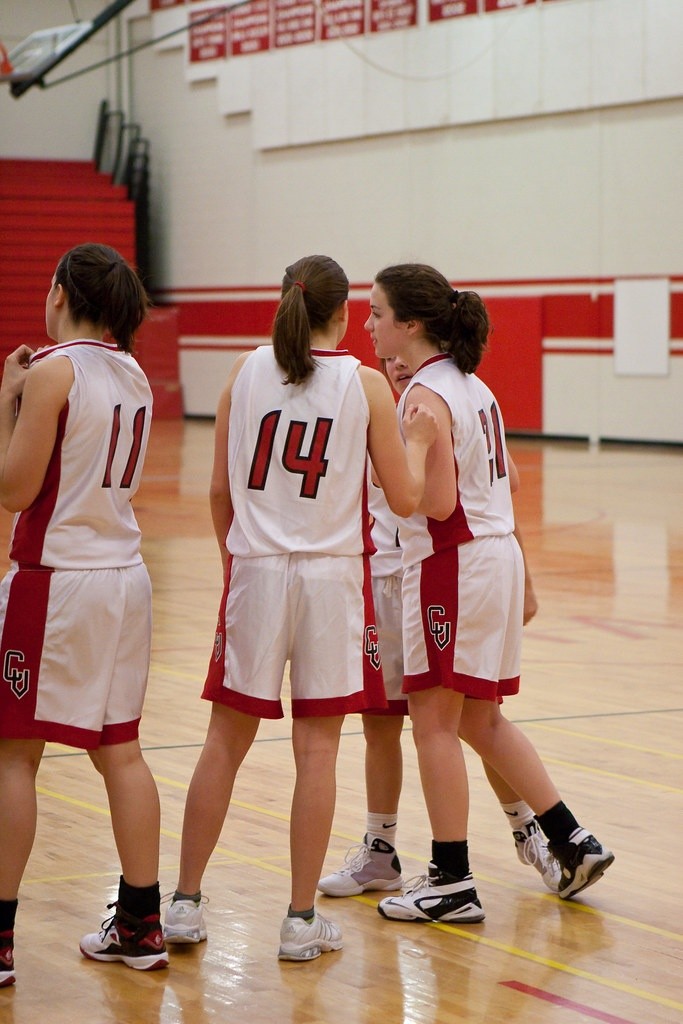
<box><xmin>160</xmin><ymin>891</ymin><xmax>212</xmax><ymax>943</ymax></box>
<box><xmin>540</xmin><ymin>827</ymin><xmax>615</xmax><ymax>899</ymax></box>
<box><xmin>512</xmin><ymin>819</ymin><xmax>564</xmax><ymax>891</ymax></box>
<box><xmin>316</xmin><ymin>832</ymin><xmax>405</xmax><ymax>895</ymax></box>
<box><xmin>0</xmin><ymin>930</ymin><xmax>17</xmax><ymax>987</ymax></box>
<box><xmin>79</xmin><ymin>900</ymin><xmax>170</xmax><ymax>970</ymax></box>
<box><xmin>277</xmin><ymin>911</ymin><xmax>343</xmax><ymax>960</ymax></box>
<box><xmin>377</xmin><ymin>860</ymin><xmax>485</xmax><ymax>922</ymax></box>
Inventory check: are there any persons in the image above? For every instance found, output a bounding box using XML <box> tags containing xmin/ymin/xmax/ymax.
<box><xmin>365</xmin><ymin>265</ymin><xmax>615</xmax><ymax>925</ymax></box>
<box><xmin>0</xmin><ymin>244</ymin><xmax>169</xmax><ymax>991</ymax></box>
<box><xmin>318</xmin><ymin>351</ymin><xmax>562</xmax><ymax>896</ymax></box>
<box><xmin>162</xmin><ymin>255</ymin><xmax>439</xmax><ymax>960</ymax></box>
